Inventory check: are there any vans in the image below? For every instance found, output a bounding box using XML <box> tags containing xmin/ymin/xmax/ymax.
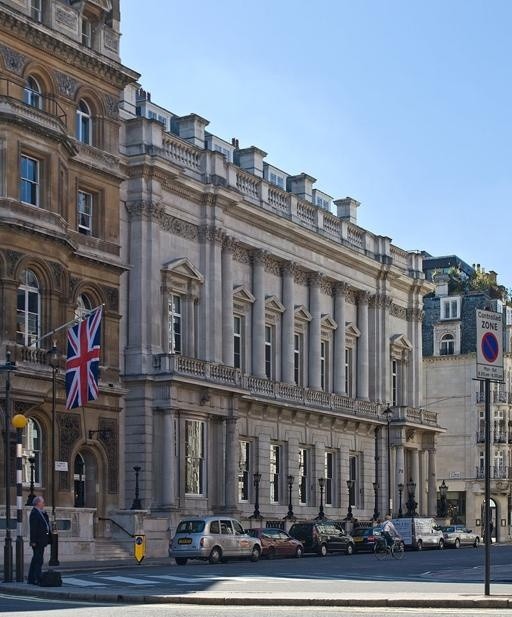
<box><xmin>388</xmin><ymin>517</ymin><xmax>446</xmax><ymax>551</ymax></box>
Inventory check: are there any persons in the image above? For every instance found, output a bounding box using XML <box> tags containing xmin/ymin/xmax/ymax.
<box><xmin>380</xmin><ymin>514</ymin><xmax>399</xmax><ymax>552</ymax></box>
<box><xmin>26</xmin><ymin>496</ymin><xmax>52</xmax><ymax>586</ymax></box>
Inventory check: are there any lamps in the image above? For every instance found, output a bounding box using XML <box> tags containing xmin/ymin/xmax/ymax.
<box><xmin>89</xmin><ymin>429</ymin><xmax>112</xmax><ymax>439</ymax></box>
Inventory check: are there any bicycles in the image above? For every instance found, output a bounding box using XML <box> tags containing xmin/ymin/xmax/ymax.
<box><xmin>372</xmin><ymin>532</ymin><xmax>405</xmax><ymax>561</ymax></box>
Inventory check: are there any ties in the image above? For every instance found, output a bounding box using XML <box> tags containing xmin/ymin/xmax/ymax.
<box><xmin>41</xmin><ymin>512</ymin><xmax>50</xmax><ymax>527</ymax></box>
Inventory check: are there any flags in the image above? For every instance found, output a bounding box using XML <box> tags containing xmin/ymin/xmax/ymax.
<box><xmin>64</xmin><ymin>308</ymin><xmax>103</xmax><ymax>412</ymax></box>
<box><xmin>132</xmin><ymin>535</ymin><xmax>147</xmax><ymax>566</ymax></box>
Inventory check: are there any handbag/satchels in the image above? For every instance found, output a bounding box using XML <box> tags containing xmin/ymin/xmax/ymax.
<box><xmin>39</xmin><ymin>573</ymin><xmax>62</xmax><ymax>587</ymax></box>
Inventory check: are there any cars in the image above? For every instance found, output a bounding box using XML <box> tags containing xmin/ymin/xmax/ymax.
<box><xmin>348</xmin><ymin>526</ymin><xmax>405</xmax><ymax>553</ymax></box>
<box><xmin>168</xmin><ymin>517</ymin><xmax>263</xmax><ymax>565</ymax></box>
<box><xmin>244</xmin><ymin>528</ymin><xmax>306</xmax><ymax>559</ymax></box>
<box><xmin>288</xmin><ymin>522</ymin><xmax>356</xmax><ymax>557</ymax></box>
<box><xmin>438</xmin><ymin>525</ymin><xmax>481</xmax><ymax>549</ymax></box>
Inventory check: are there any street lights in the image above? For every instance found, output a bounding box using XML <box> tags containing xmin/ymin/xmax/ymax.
<box><xmin>436</xmin><ymin>479</ymin><xmax>450</xmax><ymax>517</ymax></box>
<box><xmin>404</xmin><ymin>475</ymin><xmax>420</xmax><ymax>518</ymax></box>
<box><xmin>248</xmin><ymin>471</ymin><xmax>265</xmax><ymax>518</ymax></box>
<box><xmin>311</xmin><ymin>476</ymin><xmax>332</xmax><ymax>521</ymax></box>
<box><xmin>381</xmin><ymin>405</ymin><xmax>395</xmax><ymax>518</ymax></box>
<box><xmin>343</xmin><ymin>479</ymin><xmax>358</xmax><ymax>521</ymax></box>
<box><xmin>368</xmin><ymin>482</ymin><xmax>381</xmax><ymax>522</ymax></box>
<box><xmin>10</xmin><ymin>413</ymin><xmax>29</xmax><ymax>584</ymax></box>
<box><xmin>24</xmin><ymin>453</ymin><xmax>40</xmax><ymax>505</ymax></box>
<box><xmin>126</xmin><ymin>462</ymin><xmax>143</xmax><ymax>509</ymax></box>
<box><xmin>396</xmin><ymin>483</ymin><xmax>405</xmax><ymax>518</ymax></box>
<box><xmin>45</xmin><ymin>335</ymin><xmax>62</xmax><ymax>532</ymax></box>
<box><xmin>283</xmin><ymin>473</ymin><xmax>298</xmax><ymax>519</ymax></box>
